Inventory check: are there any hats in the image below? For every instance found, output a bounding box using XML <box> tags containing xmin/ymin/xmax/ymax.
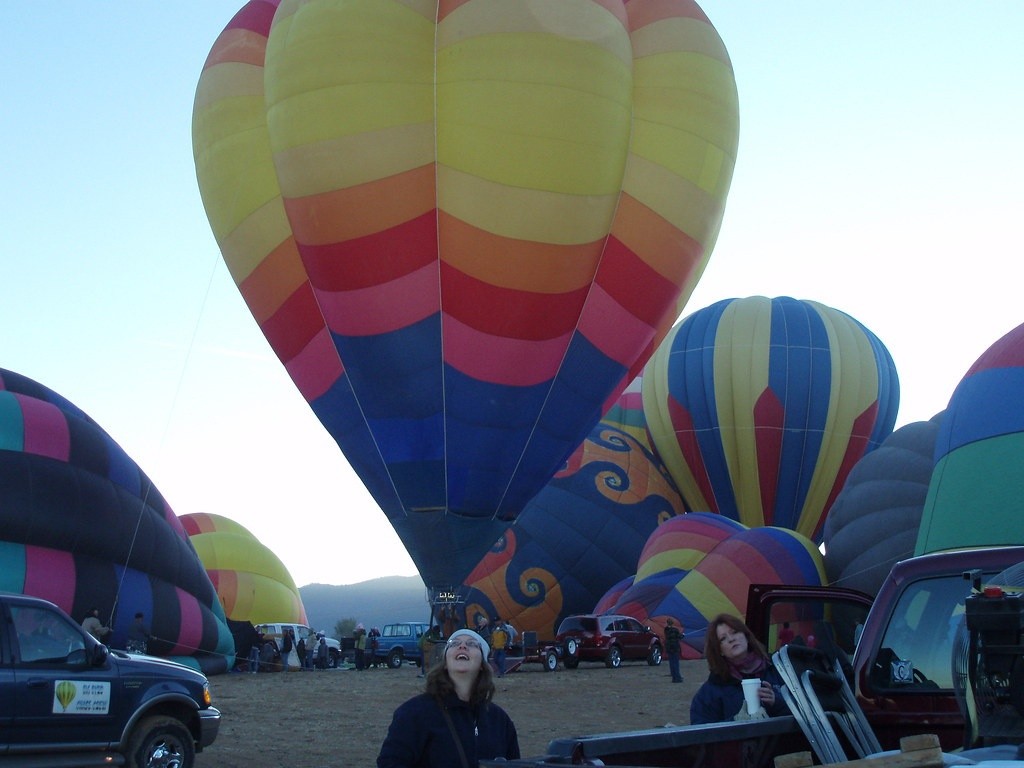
<box><xmin>494</xmin><ymin>616</ymin><xmax>500</xmax><ymax>621</ymax></box>
<box><xmin>445</xmin><ymin>629</ymin><xmax>490</xmax><ymax>664</ymax></box>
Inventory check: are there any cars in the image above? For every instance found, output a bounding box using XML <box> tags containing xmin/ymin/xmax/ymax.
<box><xmin>252</xmin><ymin>622</ymin><xmax>342</xmax><ymax>669</ymax></box>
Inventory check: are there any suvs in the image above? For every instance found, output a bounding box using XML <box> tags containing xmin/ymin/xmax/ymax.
<box><xmin>0</xmin><ymin>589</ymin><xmax>224</xmax><ymax>768</ymax></box>
<box><xmin>554</xmin><ymin>612</ymin><xmax>664</xmax><ymax>669</ymax></box>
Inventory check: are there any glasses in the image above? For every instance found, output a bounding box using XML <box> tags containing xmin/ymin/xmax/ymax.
<box><xmin>447</xmin><ymin>639</ymin><xmax>480</xmax><ymax>649</ymax></box>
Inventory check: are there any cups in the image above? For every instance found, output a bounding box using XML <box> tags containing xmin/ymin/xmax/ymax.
<box><xmin>741</xmin><ymin>678</ymin><xmax>762</xmax><ymax>715</ymax></box>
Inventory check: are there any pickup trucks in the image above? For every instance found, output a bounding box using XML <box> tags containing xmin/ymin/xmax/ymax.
<box><xmin>477</xmin><ymin>543</ymin><xmax>1024</xmax><ymax>768</ymax></box>
<box><xmin>340</xmin><ymin>621</ymin><xmax>434</xmax><ymax>669</ymax></box>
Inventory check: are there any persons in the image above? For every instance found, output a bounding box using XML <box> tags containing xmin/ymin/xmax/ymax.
<box><xmin>263</xmin><ymin>640</ymin><xmax>280</xmax><ymax>671</ymax></box>
<box><xmin>289</xmin><ymin>629</ymin><xmax>295</xmax><ymax>640</ymax></box>
<box><xmin>296</xmin><ymin>639</ymin><xmax>307</xmax><ymax>671</ymax></box>
<box><xmin>438</xmin><ymin>605</ymin><xmax>461</xmax><ymax>638</ymax></box>
<box><xmin>305</xmin><ymin>628</ymin><xmax>317</xmax><ymax>671</ymax></box>
<box><xmin>689</xmin><ymin>612</ymin><xmax>791</xmax><ymax>725</ymax></box>
<box><xmin>778</xmin><ymin>622</ymin><xmax>806</xmax><ymax>646</ymax></box>
<box><xmin>280</xmin><ymin>629</ymin><xmax>292</xmax><ymax>673</ymax></box>
<box><xmin>81</xmin><ymin>607</ymin><xmax>109</xmax><ymax>640</ymax></box>
<box><xmin>815</xmin><ymin>622</ymin><xmax>834</xmax><ymax>649</ymax></box>
<box><xmin>317</xmin><ymin>638</ymin><xmax>328</xmax><ymax>669</ymax></box>
<box><xmin>416</xmin><ymin>625</ymin><xmax>441</xmax><ymax>675</ymax></box>
<box><xmin>664</xmin><ymin>618</ymin><xmax>684</xmax><ymax>683</ymax></box>
<box><xmin>127</xmin><ymin>612</ymin><xmax>149</xmax><ymax>653</ymax></box>
<box><xmin>247</xmin><ymin>625</ymin><xmax>262</xmax><ymax>673</ymax></box>
<box><xmin>332</xmin><ymin>638</ymin><xmax>354</xmax><ymax>668</ymax></box>
<box><xmin>353</xmin><ymin>629</ymin><xmax>367</xmax><ymax>671</ymax></box>
<box><xmin>376</xmin><ymin>629</ymin><xmax>520</xmax><ymax>768</ymax></box>
<box><xmin>368</xmin><ymin>626</ymin><xmax>380</xmax><ymax>637</ymax></box>
<box><xmin>473</xmin><ymin>617</ymin><xmax>518</xmax><ymax>678</ymax></box>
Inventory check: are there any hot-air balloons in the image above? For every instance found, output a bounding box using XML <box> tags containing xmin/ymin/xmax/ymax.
<box><xmin>591</xmin><ymin>510</ymin><xmax>835</xmax><ymax>665</ymax></box>
<box><xmin>821</xmin><ymin>408</ymin><xmax>946</xmax><ymax>630</ymax></box>
<box><xmin>186</xmin><ymin>2</ymin><xmax>744</xmax><ymax>664</ymax></box>
<box><xmin>177</xmin><ymin>512</ymin><xmax>310</xmax><ymax>629</ymax></box>
<box><xmin>0</xmin><ymin>366</ymin><xmax>238</xmax><ymax>677</ymax></box>
<box><xmin>907</xmin><ymin>320</ymin><xmax>1023</xmax><ymax>559</ymax></box>
<box><xmin>640</xmin><ymin>294</ymin><xmax>901</xmax><ymax>549</ymax></box>
<box><xmin>428</xmin><ymin>368</ymin><xmax>690</xmax><ymax>658</ymax></box>
